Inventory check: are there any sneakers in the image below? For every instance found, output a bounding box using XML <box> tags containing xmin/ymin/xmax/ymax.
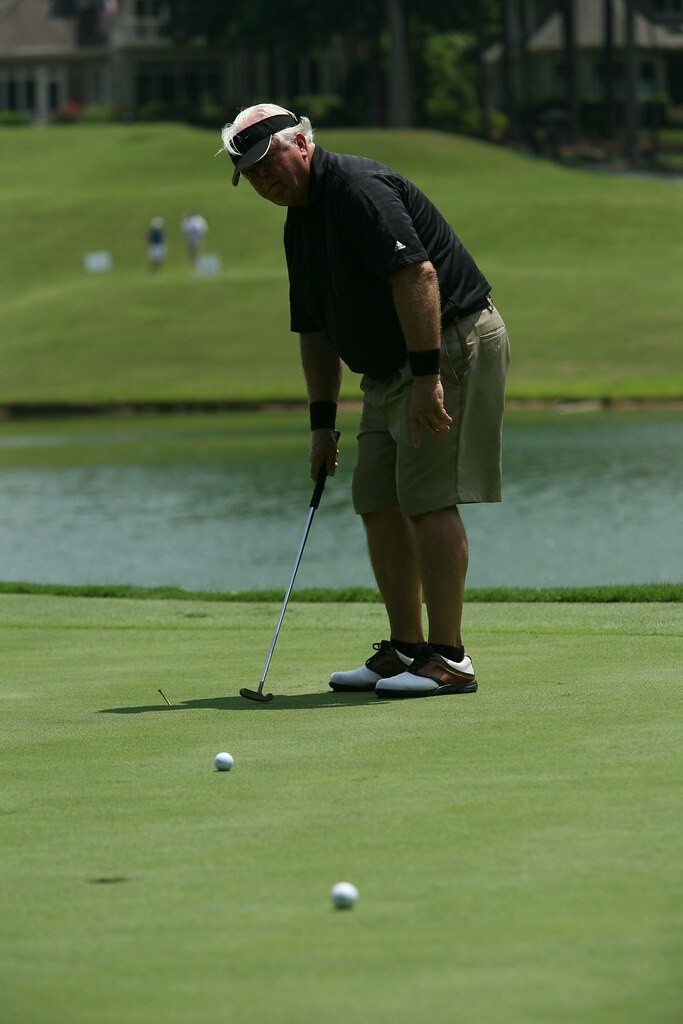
<box><xmin>328</xmin><ymin>640</ymin><xmax>479</xmax><ymax>698</ymax></box>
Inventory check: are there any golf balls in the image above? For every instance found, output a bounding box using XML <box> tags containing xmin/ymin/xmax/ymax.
<box><xmin>330</xmin><ymin>881</ymin><xmax>360</xmax><ymax>907</ymax></box>
<box><xmin>215</xmin><ymin>752</ymin><xmax>234</xmax><ymax>770</ymax></box>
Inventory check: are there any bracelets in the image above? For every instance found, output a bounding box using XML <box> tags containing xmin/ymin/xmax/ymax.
<box><xmin>309</xmin><ymin>401</ymin><xmax>338</xmax><ymax>430</ymax></box>
<box><xmin>407</xmin><ymin>347</ymin><xmax>441</xmax><ymax>377</ymax></box>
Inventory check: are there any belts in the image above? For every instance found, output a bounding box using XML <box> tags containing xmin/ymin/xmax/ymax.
<box><xmin>459</xmin><ymin>297</ymin><xmax>493</xmax><ymax>320</ymax></box>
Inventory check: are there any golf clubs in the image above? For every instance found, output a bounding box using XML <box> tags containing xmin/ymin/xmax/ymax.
<box><xmin>239</xmin><ymin>432</ymin><xmax>345</xmax><ymax>702</ymax></box>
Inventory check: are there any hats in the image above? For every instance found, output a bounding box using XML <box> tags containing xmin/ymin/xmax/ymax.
<box><xmin>227</xmin><ymin>112</ymin><xmax>304</xmax><ymax>187</ymax></box>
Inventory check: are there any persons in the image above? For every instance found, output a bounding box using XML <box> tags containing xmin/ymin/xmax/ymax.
<box><xmin>220</xmin><ymin>102</ymin><xmax>509</xmax><ymax>696</ymax></box>
<box><xmin>177</xmin><ymin>210</ymin><xmax>207</xmax><ymax>273</ymax></box>
<box><xmin>147</xmin><ymin>216</ymin><xmax>165</xmax><ymax>271</ymax></box>
